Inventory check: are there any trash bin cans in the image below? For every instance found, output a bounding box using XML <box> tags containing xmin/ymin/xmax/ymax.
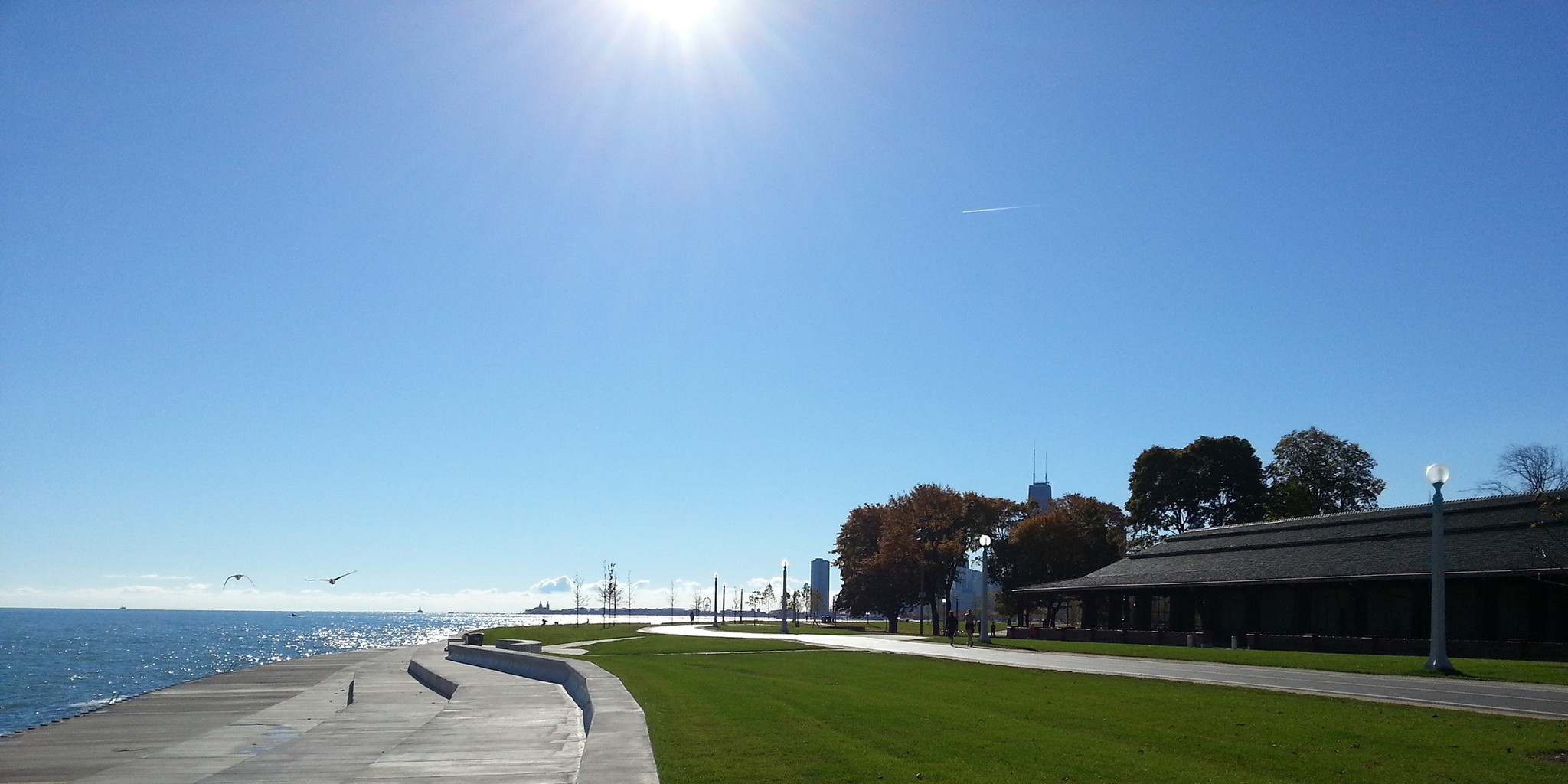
<box><xmin>826</xmin><ymin>616</ymin><xmax>831</xmax><ymax>622</ymax></box>
<box><xmin>821</xmin><ymin>616</ymin><xmax>826</xmax><ymax>623</ymax></box>
<box><xmin>464</xmin><ymin>633</ymin><xmax>484</xmax><ymax>646</ymax></box>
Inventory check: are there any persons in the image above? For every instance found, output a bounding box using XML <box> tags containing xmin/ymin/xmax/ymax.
<box><xmin>977</xmin><ymin>618</ymin><xmax>981</xmax><ymax>634</ymax></box>
<box><xmin>990</xmin><ymin>622</ymin><xmax>996</xmax><ymax>637</ymax></box>
<box><xmin>690</xmin><ymin>611</ymin><xmax>695</xmax><ymax>623</ymax></box>
<box><xmin>944</xmin><ymin>610</ymin><xmax>959</xmax><ymax>647</ymax></box>
<box><xmin>963</xmin><ymin>609</ymin><xmax>977</xmax><ymax>647</ymax></box>
<box><xmin>813</xmin><ymin>612</ymin><xmax>820</xmax><ymax>627</ymax></box>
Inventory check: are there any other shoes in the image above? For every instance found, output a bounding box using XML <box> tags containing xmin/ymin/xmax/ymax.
<box><xmin>970</xmin><ymin>641</ymin><xmax>973</xmax><ymax>647</ymax></box>
<box><xmin>966</xmin><ymin>642</ymin><xmax>969</xmax><ymax>646</ymax></box>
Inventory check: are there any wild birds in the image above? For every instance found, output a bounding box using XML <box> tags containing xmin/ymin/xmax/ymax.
<box><xmin>223</xmin><ymin>574</ymin><xmax>256</xmax><ymax>590</ymax></box>
<box><xmin>304</xmin><ymin>570</ymin><xmax>358</xmax><ymax>584</ymax></box>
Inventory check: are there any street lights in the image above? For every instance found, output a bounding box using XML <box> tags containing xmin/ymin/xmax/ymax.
<box><xmin>1424</xmin><ymin>462</ymin><xmax>1454</xmax><ymax>674</ymax></box>
<box><xmin>978</xmin><ymin>535</ymin><xmax>992</xmax><ymax>645</ymax></box>
<box><xmin>942</xmin><ymin>598</ymin><xmax>946</xmax><ymax>632</ymax></box>
<box><xmin>780</xmin><ymin>559</ymin><xmax>789</xmax><ymax>634</ymax></box>
<box><xmin>721</xmin><ymin>582</ymin><xmax>726</xmax><ymax>625</ymax></box>
<box><xmin>713</xmin><ymin>572</ymin><xmax>718</xmax><ymax>627</ymax></box>
<box><xmin>739</xmin><ymin>585</ymin><xmax>743</xmax><ymax>624</ymax></box>
